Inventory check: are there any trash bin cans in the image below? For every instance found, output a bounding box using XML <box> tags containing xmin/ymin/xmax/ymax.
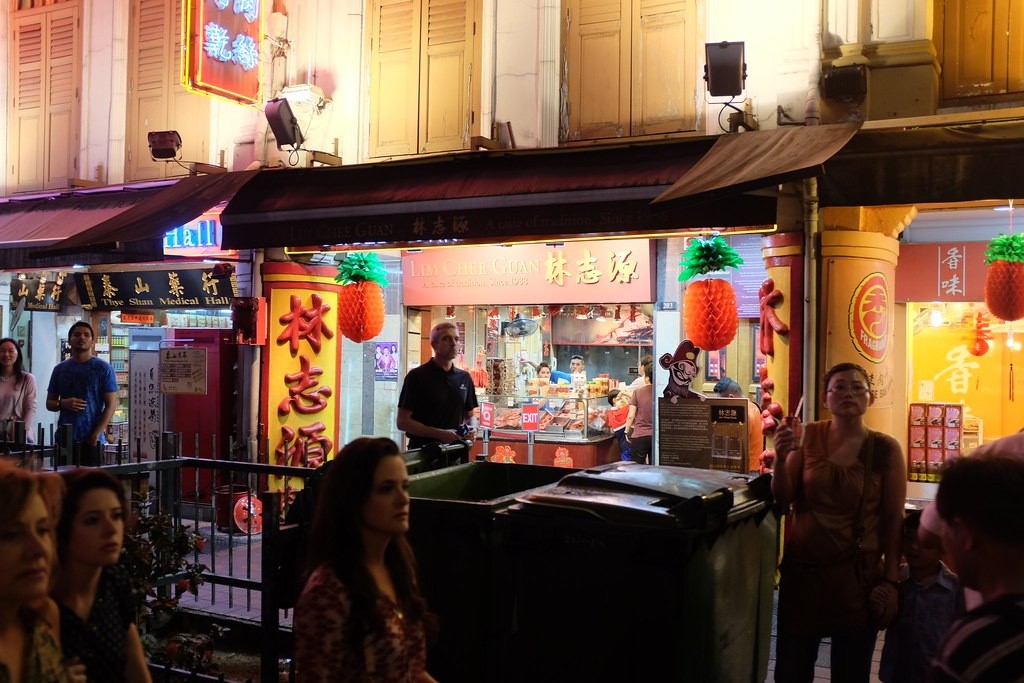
<box><xmin>494</xmin><ymin>461</ymin><xmax>776</xmax><ymax>683</ymax></box>
<box><xmin>407</xmin><ymin>455</ymin><xmax>585</xmax><ymax>683</ymax></box>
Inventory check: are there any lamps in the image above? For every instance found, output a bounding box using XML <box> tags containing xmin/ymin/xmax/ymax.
<box><xmin>488</xmin><ymin>304</ymin><xmax>637</xmax><ymax>324</ymax></box>
<box><xmin>823</xmin><ymin>62</ymin><xmax>868</xmax><ymax>126</ymax></box>
<box><xmin>445</xmin><ymin>305</ymin><xmax>457</xmax><ymax>320</ymax></box>
<box><xmin>265</xmin><ymin>98</ymin><xmax>343</xmax><ymax>169</ymax></box>
<box><xmin>148</xmin><ymin>130</ymin><xmax>227</xmax><ymax>177</ymax></box>
<box><xmin>703</xmin><ymin>40</ymin><xmax>759</xmax><ymax>133</ymax></box>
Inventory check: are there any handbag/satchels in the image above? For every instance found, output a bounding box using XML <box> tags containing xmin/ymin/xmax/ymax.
<box><xmin>832</xmin><ymin>550</ymin><xmax>883</xmax><ymax>626</ymax></box>
<box><xmin>0</xmin><ymin>431</ymin><xmax>11</xmax><ymax>453</ymax></box>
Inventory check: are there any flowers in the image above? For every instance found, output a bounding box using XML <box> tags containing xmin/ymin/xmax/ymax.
<box><xmin>115</xmin><ymin>486</ymin><xmax>231</xmax><ymax>672</ymax></box>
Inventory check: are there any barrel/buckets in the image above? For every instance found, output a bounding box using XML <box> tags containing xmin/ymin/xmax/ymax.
<box><xmin>216</xmin><ymin>484</ymin><xmax>254</xmax><ymax>533</ymax></box>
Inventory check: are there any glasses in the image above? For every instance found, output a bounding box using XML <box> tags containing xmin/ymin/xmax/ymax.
<box><xmin>826</xmin><ymin>384</ymin><xmax>870</xmax><ymax>396</ymax></box>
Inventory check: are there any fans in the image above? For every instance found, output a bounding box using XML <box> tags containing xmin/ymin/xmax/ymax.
<box><xmin>502</xmin><ymin>313</ymin><xmax>538</xmax><ymax>339</ymax></box>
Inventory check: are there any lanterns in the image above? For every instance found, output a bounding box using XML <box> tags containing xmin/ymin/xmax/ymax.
<box><xmin>961</xmin><ymin>232</ymin><xmax>1024</xmax><ymax>401</ymax></box>
<box><xmin>678</xmin><ymin>233</ymin><xmax>744</xmax><ymax>351</ymax></box>
<box><xmin>334</xmin><ymin>250</ymin><xmax>390</xmax><ymax>343</ymax></box>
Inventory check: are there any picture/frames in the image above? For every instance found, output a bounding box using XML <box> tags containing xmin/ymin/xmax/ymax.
<box><xmin>752</xmin><ymin>324</ymin><xmax>767</xmax><ymax>382</ymax></box>
<box><xmin>704</xmin><ymin>348</ymin><xmax>720</xmax><ymax>381</ymax></box>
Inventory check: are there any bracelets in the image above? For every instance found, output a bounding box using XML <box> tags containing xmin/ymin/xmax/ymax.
<box><xmin>475</xmin><ymin>426</ymin><xmax>479</xmax><ymax>432</ymax></box>
<box><xmin>883</xmin><ymin>577</ymin><xmax>902</xmax><ymax>588</ymax></box>
<box><xmin>57</xmin><ymin>400</ymin><xmax>60</xmax><ymax>409</ymax></box>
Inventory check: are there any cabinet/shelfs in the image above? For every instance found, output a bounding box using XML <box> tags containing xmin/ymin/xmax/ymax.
<box><xmin>110</xmin><ymin>334</ymin><xmax>129</xmax><ymax>426</ymax></box>
<box><xmin>961</xmin><ymin>418</ymin><xmax>983</xmax><ymax>449</ymax></box>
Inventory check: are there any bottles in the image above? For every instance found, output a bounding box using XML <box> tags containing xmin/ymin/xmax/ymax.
<box><xmin>116</xmin><ymin>373</ymin><xmax>129</xmax><ymax>398</ymax></box>
<box><xmin>112</xmin><ymin>361</ymin><xmax>124</xmax><ymax>371</ymax></box>
<box><xmin>587</xmin><ymin>382</ymin><xmax>600</xmax><ymax>398</ymax></box>
<box><xmin>558</xmin><ymin>382</ymin><xmax>569</xmax><ymax>398</ymax></box>
<box><xmin>97</xmin><ymin>336</ymin><xmax>125</xmax><ymax>346</ymax></box>
<box><xmin>111</xmin><ymin>350</ymin><xmax>127</xmax><ymax>360</ymax></box>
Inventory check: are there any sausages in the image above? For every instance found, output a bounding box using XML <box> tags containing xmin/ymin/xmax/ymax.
<box><xmin>469</xmin><ymin>369</ymin><xmax>488</xmax><ymax>387</ymax></box>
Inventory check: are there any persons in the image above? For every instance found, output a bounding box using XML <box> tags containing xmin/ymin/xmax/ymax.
<box><xmin>516</xmin><ymin>355</ymin><xmax>588</xmax><ymax>411</ymax></box>
<box><xmin>0</xmin><ymin>338</ymin><xmax>38</xmax><ymax>452</ymax></box>
<box><xmin>772</xmin><ymin>362</ymin><xmax>1024</xmax><ymax>683</ymax></box>
<box><xmin>45</xmin><ymin>322</ymin><xmax>119</xmax><ymax>466</ymax></box>
<box><xmin>374</xmin><ymin>345</ymin><xmax>397</xmax><ymax>373</ymax></box>
<box><xmin>0</xmin><ymin>456</ymin><xmax>154</xmax><ymax>683</ymax></box>
<box><xmin>292</xmin><ymin>437</ymin><xmax>439</xmax><ymax>683</ymax></box>
<box><xmin>397</xmin><ymin>323</ymin><xmax>479</xmax><ymax>449</ymax></box>
<box><xmin>605</xmin><ymin>354</ymin><xmax>653</xmax><ymax>464</ymax></box>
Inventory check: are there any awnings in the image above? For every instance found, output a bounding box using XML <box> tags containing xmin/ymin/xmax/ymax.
<box><xmin>218</xmin><ymin>173</ymin><xmax>779</xmax><ymax>250</ymax></box>
<box><xmin>0</xmin><ymin>170</ymin><xmax>257</xmax><ymax>251</ymax></box>
<box><xmin>815</xmin><ymin>140</ymin><xmax>1024</xmax><ymax>212</ymax></box>
